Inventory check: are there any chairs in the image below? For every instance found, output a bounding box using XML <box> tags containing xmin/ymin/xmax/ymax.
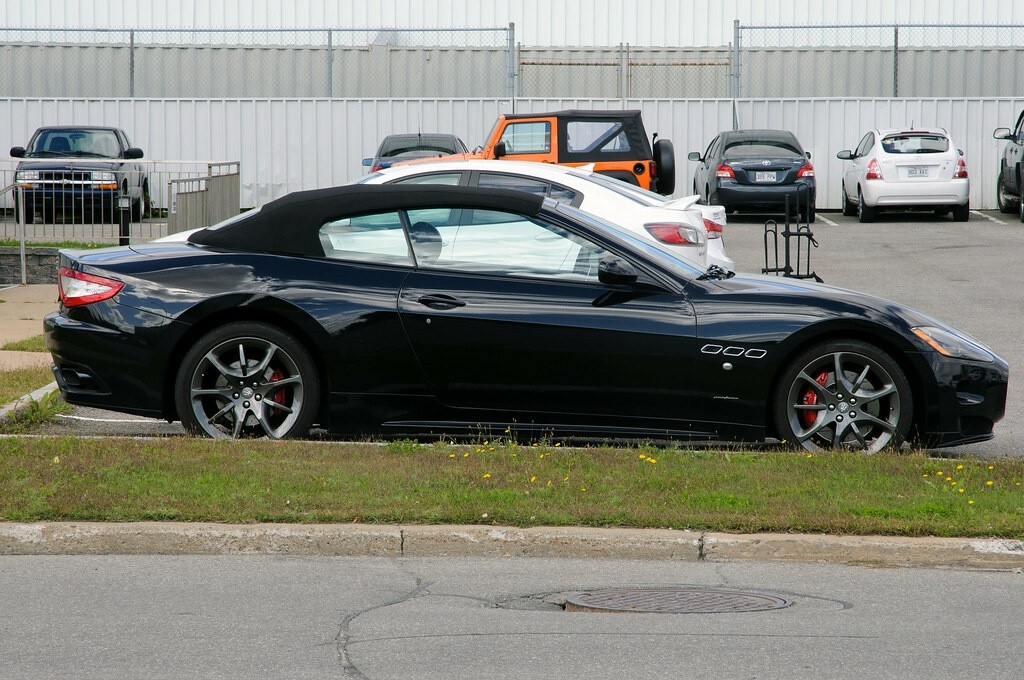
<box><xmin>920</xmin><ymin>138</ymin><xmax>936</xmax><ymax>150</ymax></box>
<box><xmin>92</xmin><ymin>137</ymin><xmax>115</xmax><ymax>156</ymax></box>
<box><xmin>49</xmin><ymin>137</ymin><xmax>71</xmax><ymax>151</ymax></box>
<box><xmin>409</xmin><ymin>221</ymin><xmax>443</xmax><ymax>267</ymax></box>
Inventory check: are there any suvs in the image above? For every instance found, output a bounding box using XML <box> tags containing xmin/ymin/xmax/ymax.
<box><xmin>373</xmin><ymin>108</ymin><xmax>676</xmax><ymax>197</ymax></box>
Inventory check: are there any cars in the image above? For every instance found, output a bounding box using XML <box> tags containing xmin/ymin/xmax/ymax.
<box><xmin>688</xmin><ymin>129</ymin><xmax>816</xmax><ymax>223</ymax></box>
<box><xmin>992</xmin><ymin>110</ymin><xmax>1024</xmax><ymax>221</ymax></box>
<box><xmin>836</xmin><ymin>126</ymin><xmax>971</xmax><ymax>221</ymax></box>
<box><xmin>151</xmin><ymin>158</ymin><xmax>733</xmax><ymax>280</ymax></box>
<box><xmin>361</xmin><ymin>132</ymin><xmax>468</xmax><ymax>172</ymax></box>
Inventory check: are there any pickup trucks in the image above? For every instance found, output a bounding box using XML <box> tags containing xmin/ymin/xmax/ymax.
<box><xmin>10</xmin><ymin>125</ymin><xmax>147</xmax><ymax>221</ymax></box>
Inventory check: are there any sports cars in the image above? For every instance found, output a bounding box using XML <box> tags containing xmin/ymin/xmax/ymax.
<box><xmin>42</xmin><ymin>186</ymin><xmax>1009</xmax><ymax>454</ymax></box>
